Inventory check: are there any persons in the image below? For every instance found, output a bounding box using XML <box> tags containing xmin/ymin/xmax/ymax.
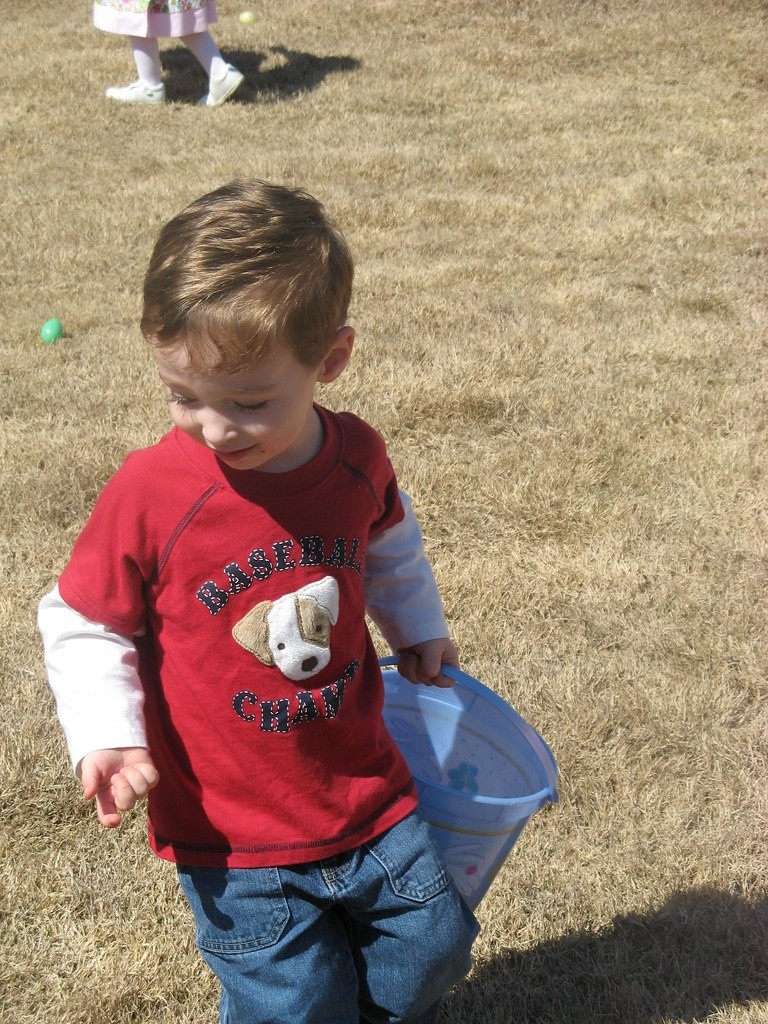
<box><xmin>93</xmin><ymin>0</ymin><xmax>244</xmax><ymax>108</ymax></box>
<box><xmin>36</xmin><ymin>178</ymin><xmax>481</xmax><ymax>1024</ymax></box>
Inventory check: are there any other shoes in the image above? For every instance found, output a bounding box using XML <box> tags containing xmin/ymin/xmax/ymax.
<box><xmin>105</xmin><ymin>81</ymin><xmax>166</xmax><ymax>104</ymax></box>
<box><xmin>197</xmin><ymin>63</ymin><xmax>245</xmax><ymax>107</ymax></box>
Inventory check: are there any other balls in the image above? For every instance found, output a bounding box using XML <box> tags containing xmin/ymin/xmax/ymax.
<box><xmin>41</xmin><ymin>316</ymin><xmax>63</xmax><ymax>342</ymax></box>
<box><xmin>238</xmin><ymin>10</ymin><xmax>257</xmax><ymax>25</ymax></box>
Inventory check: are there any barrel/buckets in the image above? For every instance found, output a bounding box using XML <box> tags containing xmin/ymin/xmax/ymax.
<box><xmin>379</xmin><ymin>656</ymin><xmax>559</xmax><ymax>912</ymax></box>
